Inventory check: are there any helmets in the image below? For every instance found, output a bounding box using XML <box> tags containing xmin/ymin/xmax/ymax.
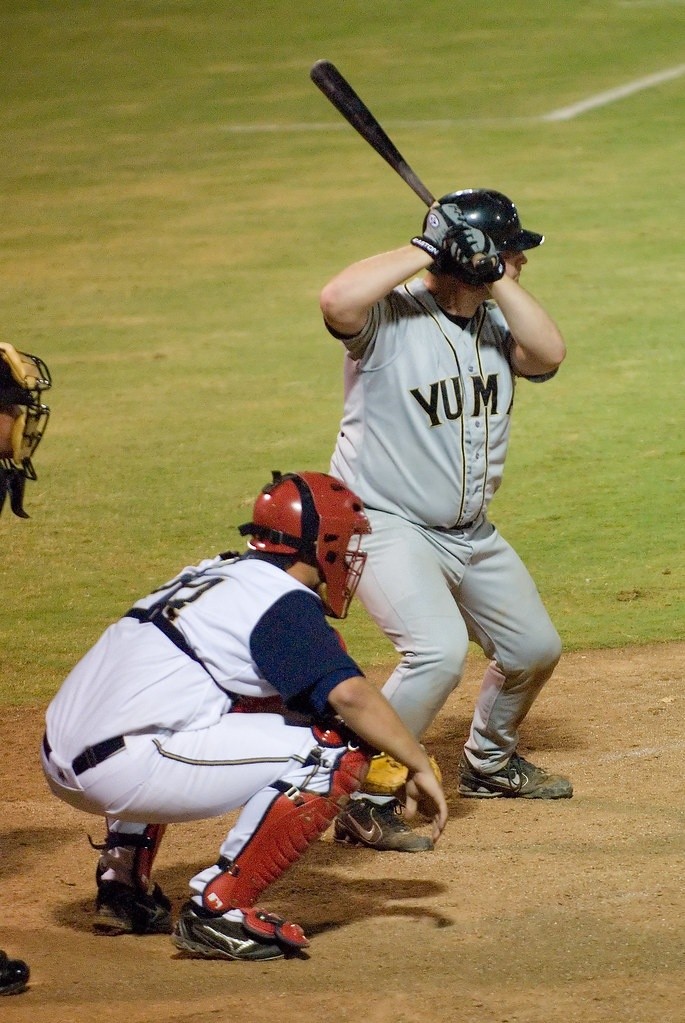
<box><xmin>0</xmin><ymin>341</ymin><xmax>33</xmax><ymax>407</ymax></box>
<box><xmin>422</xmin><ymin>187</ymin><xmax>545</xmax><ymax>283</ymax></box>
<box><xmin>247</xmin><ymin>470</ymin><xmax>373</xmax><ymax>619</ymax></box>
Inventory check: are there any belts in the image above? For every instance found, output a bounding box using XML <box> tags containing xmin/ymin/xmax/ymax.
<box><xmin>433</xmin><ymin>520</ymin><xmax>473</xmax><ymax>532</ymax></box>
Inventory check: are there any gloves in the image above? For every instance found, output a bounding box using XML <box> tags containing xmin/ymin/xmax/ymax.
<box><xmin>446</xmin><ymin>223</ymin><xmax>506</xmax><ymax>282</ymax></box>
<box><xmin>410</xmin><ymin>203</ymin><xmax>466</xmax><ymax>263</ymax></box>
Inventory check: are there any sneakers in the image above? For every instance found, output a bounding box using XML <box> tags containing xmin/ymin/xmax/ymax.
<box><xmin>175</xmin><ymin>900</ymin><xmax>296</xmax><ymax>961</ymax></box>
<box><xmin>332</xmin><ymin>789</ymin><xmax>434</xmax><ymax>853</ymax></box>
<box><xmin>456</xmin><ymin>751</ymin><xmax>573</xmax><ymax>799</ymax></box>
<box><xmin>86</xmin><ymin>880</ymin><xmax>176</xmax><ymax>936</ymax></box>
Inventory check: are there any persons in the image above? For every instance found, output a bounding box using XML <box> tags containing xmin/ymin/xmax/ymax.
<box><xmin>0</xmin><ymin>339</ymin><xmax>53</xmax><ymax>994</ymax></box>
<box><xmin>316</xmin><ymin>188</ymin><xmax>573</xmax><ymax>854</ymax></box>
<box><xmin>39</xmin><ymin>470</ymin><xmax>449</xmax><ymax>963</ymax></box>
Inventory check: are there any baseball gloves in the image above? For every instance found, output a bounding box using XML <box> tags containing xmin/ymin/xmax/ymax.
<box><xmin>333</xmin><ymin>797</ymin><xmax>436</xmax><ymax>856</ymax></box>
<box><xmin>0</xmin><ymin>342</ymin><xmax>54</xmax><ymax>521</ymax></box>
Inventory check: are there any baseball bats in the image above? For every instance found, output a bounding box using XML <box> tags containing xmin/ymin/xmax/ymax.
<box><xmin>307</xmin><ymin>57</ymin><xmax>495</xmax><ymax>276</ymax></box>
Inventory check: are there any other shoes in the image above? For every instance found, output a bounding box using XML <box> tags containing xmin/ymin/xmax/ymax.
<box><xmin>0</xmin><ymin>950</ymin><xmax>30</xmax><ymax>996</ymax></box>
